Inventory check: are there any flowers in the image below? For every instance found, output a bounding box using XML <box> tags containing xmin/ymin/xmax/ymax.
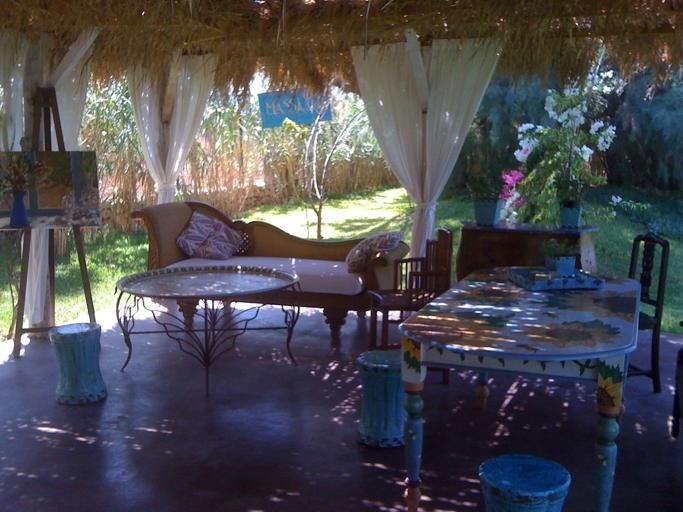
<box><xmin>518</xmin><ymin>47</ymin><xmax>627</xmax><ymax>203</ymax></box>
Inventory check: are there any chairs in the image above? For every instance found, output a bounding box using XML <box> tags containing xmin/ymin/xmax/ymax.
<box><xmin>368</xmin><ymin>229</ymin><xmax>452</xmax><ymax>351</ymax></box>
<box><xmin>627</xmin><ymin>233</ymin><xmax>669</xmax><ymax>393</ymax></box>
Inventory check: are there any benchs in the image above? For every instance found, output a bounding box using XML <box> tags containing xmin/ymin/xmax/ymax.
<box><xmin>124</xmin><ymin>199</ymin><xmax>408</xmax><ymax>348</ymax></box>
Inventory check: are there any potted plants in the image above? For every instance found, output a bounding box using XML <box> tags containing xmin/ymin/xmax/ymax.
<box><xmin>545</xmin><ymin>238</ymin><xmax>583</xmax><ymax>274</ymax></box>
<box><xmin>458</xmin><ymin>112</ymin><xmax>511</xmax><ymax>227</ymax></box>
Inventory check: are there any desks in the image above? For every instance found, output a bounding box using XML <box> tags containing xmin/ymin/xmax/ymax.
<box><xmin>401</xmin><ymin>263</ymin><xmax>641</xmax><ymax>512</ymax></box>
<box><xmin>456</xmin><ymin>219</ymin><xmax>597</xmax><ymax>277</ymax></box>
<box><xmin>112</xmin><ymin>266</ymin><xmax>302</xmax><ymax>400</ymax></box>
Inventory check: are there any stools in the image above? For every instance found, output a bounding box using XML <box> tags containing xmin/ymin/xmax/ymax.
<box><xmin>355</xmin><ymin>352</ymin><xmax>406</xmax><ymax>450</ymax></box>
<box><xmin>49</xmin><ymin>323</ymin><xmax>107</xmax><ymax>406</ymax></box>
<box><xmin>479</xmin><ymin>454</ymin><xmax>572</xmax><ymax>512</ymax></box>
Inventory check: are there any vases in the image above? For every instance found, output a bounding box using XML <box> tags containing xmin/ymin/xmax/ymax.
<box><xmin>561</xmin><ymin>202</ymin><xmax>581</xmax><ymax>227</ymax></box>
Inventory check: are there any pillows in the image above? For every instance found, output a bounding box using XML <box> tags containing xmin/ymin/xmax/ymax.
<box><xmin>176</xmin><ymin>210</ymin><xmax>242</xmax><ymax>259</ymax></box>
<box><xmin>346</xmin><ymin>231</ymin><xmax>403</xmax><ymax>274</ymax></box>
